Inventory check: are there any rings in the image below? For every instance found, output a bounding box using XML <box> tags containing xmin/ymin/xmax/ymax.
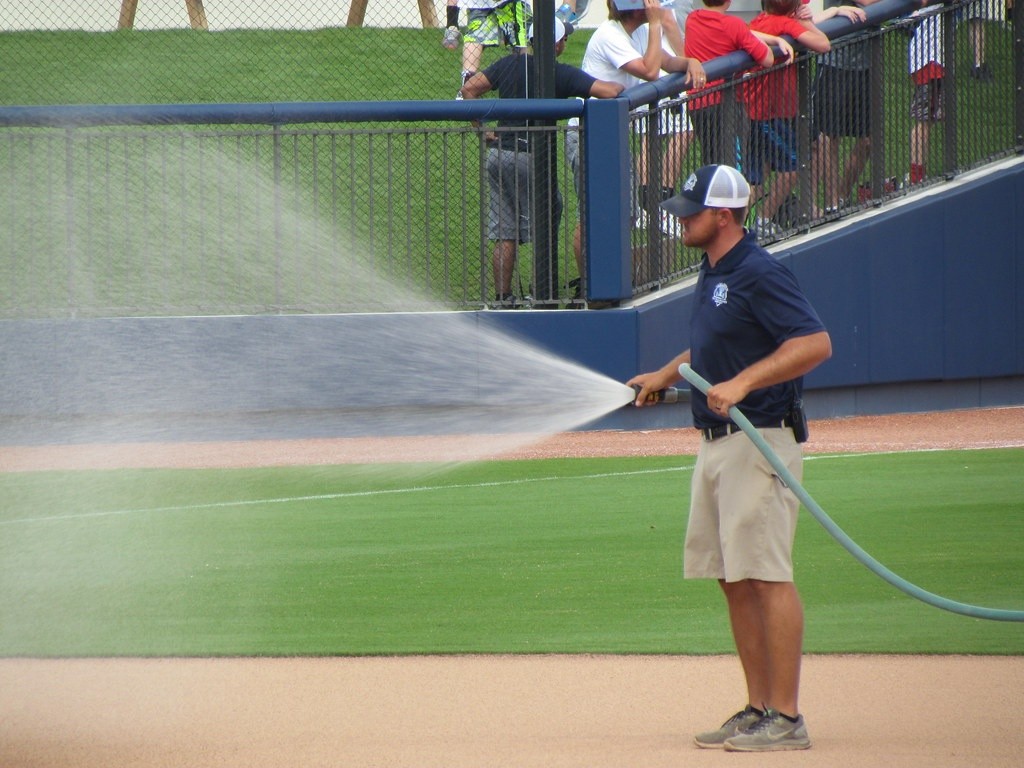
<box><xmin>716</xmin><ymin>406</ymin><xmax>721</xmax><ymax>410</ymax></box>
<box><xmin>700</xmin><ymin>78</ymin><xmax>704</xmax><ymax>80</ymax></box>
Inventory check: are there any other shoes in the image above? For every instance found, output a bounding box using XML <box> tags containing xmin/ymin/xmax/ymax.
<box><xmin>635</xmin><ymin>210</ymin><xmax>684</xmax><ymax>240</ymax></box>
<box><xmin>442</xmin><ymin>27</ymin><xmax>459</xmax><ymax>50</ymax></box>
<box><xmin>751</xmin><ymin>221</ymin><xmax>783</xmax><ymax>239</ymax></box>
<box><xmin>497</xmin><ymin>295</ymin><xmax>521</xmax><ymax>310</ymax></box>
<box><xmin>563</xmin><ymin>278</ymin><xmax>581</xmax><ymax>309</ymax></box>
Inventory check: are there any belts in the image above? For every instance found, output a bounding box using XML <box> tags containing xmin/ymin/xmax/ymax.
<box><xmin>701</xmin><ymin>415</ymin><xmax>793</xmax><ymax>441</ymax></box>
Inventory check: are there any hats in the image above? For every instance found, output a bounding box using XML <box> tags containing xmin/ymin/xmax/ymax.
<box><xmin>529</xmin><ymin>16</ymin><xmax>574</xmax><ymax>47</ymax></box>
<box><xmin>660</xmin><ymin>163</ymin><xmax>753</xmax><ymax>217</ymax></box>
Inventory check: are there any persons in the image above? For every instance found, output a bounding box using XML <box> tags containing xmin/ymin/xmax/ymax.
<box><xmin>626</xmin><ymin>164</ymin><xmax>834</xmax><ymax>751</ymax></box>
<box><xmin>441</xmin><ymin>0</ymin><xmax>1013</xmax><ymax>310</ymax></box>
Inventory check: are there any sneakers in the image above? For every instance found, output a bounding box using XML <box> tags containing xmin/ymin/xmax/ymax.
<box><xmin>693</xmin><ymin>704</ymin><xmax>768</xmax><ymax>749</ymax></box>
<box><xmin>722</xmin><ymin>702</ymin><xmax>813</xmax><ymax>751</ymax></box>
